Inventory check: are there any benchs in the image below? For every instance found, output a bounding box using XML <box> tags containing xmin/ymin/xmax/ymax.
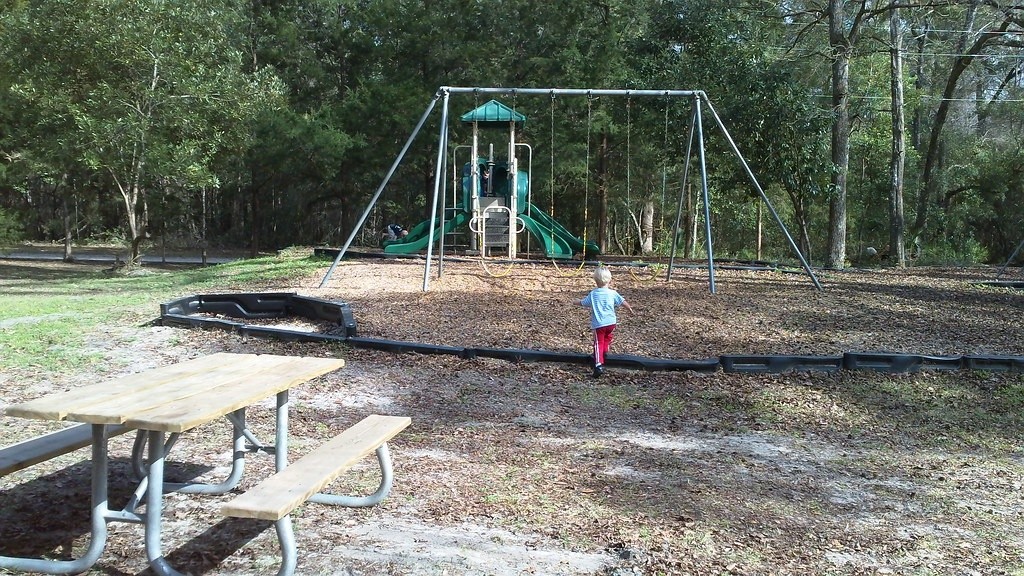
<box><xmin>221</xmin><ymin>412</ymin><xmax>411</xmax><ymax>576</ymax></box>
<box><xmin>0</xmin><ymin>423</ymin><xmax>150</xmax><ymax>575</ymax></box>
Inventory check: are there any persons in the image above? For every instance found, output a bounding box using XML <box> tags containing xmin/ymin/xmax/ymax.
<box><xmin>481</xmin><ymin>170</ymin><xmax>494</xmax><ymax>194</ymax></box>
<box><xmin>382</xmin><ymin>224</ymin><xmax>408</xmax><ymax>240</ymax></box>
<box><xmin>573</xmin><ymin>268</ymin><xmax>634</xmax><ymax>378</ymax></box>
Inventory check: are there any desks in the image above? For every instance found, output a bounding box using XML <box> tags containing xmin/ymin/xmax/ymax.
<box><xmin>4</xmin><ymin>351</ymin><xmax>344</xmax><ymax>576</ymax></box>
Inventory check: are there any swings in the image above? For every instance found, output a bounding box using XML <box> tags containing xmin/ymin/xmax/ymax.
<box><xmin>549</xmin><ymin>94</ymin><xmax>592</xmax><ymax>278</ymax></box>
<box><xmin>625</xmin><ymin>94</ymin><xmax>670</xmax><ymax>283</ymax></box>
<box><xmin>471</xmin><ymin>92</ymin><xmax>520</xmax><ymax>278</ymax></box>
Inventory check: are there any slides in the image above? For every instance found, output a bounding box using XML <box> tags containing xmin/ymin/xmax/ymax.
<box><xmin>517</xmin><ymin>200</ymin><xmax>601</xmax><ymax>260</ymax></box>
<box><xmin>382</xmin><ymin>203</ymin><xmax>471</xmax><ymax>253</ymax></box>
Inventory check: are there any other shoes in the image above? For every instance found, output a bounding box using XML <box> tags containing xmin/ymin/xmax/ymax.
<box><xmin>594</xmin><ymin>366</ymin><xmax>603</xmax><ymax>378</ymax></box>
